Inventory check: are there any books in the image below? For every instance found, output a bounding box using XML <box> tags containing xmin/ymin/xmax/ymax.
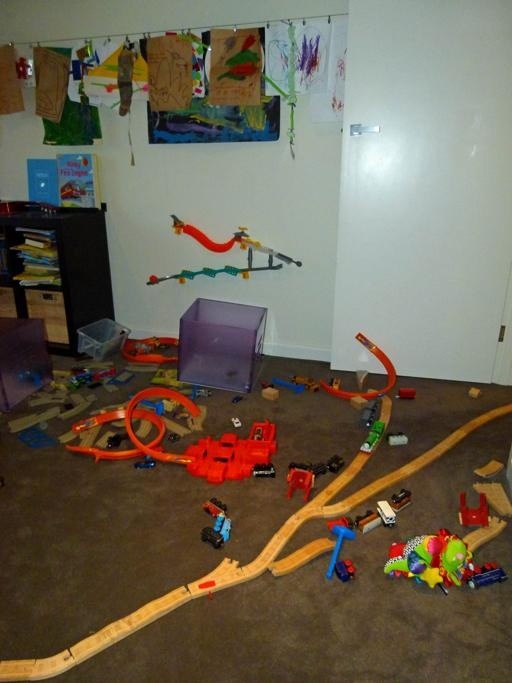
<box><xmin>12</xmin><ymin>227</ymin><xmax>61</xmax><ymax>288</ymax></box>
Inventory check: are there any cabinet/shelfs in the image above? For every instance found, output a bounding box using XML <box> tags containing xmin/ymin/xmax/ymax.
<box><xmin>1</xmin><ymin>201</ymin><xmax>117</xmax><ymax>359</ymax></box>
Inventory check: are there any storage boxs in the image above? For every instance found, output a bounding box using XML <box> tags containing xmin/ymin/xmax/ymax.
<box><xmin>76</xmin><ymin>316</ymin><xmax>131</xmax><ymax>363</ymax></box>
<box><xmin>0</xmin><ymin>316</ymin><xmax>55</xmax><ymax>410</ymax></box>
<box><xmin>174</xmin><ymin>294</ymin><xmax>269</xmax><ymax>396</ymax></box>
